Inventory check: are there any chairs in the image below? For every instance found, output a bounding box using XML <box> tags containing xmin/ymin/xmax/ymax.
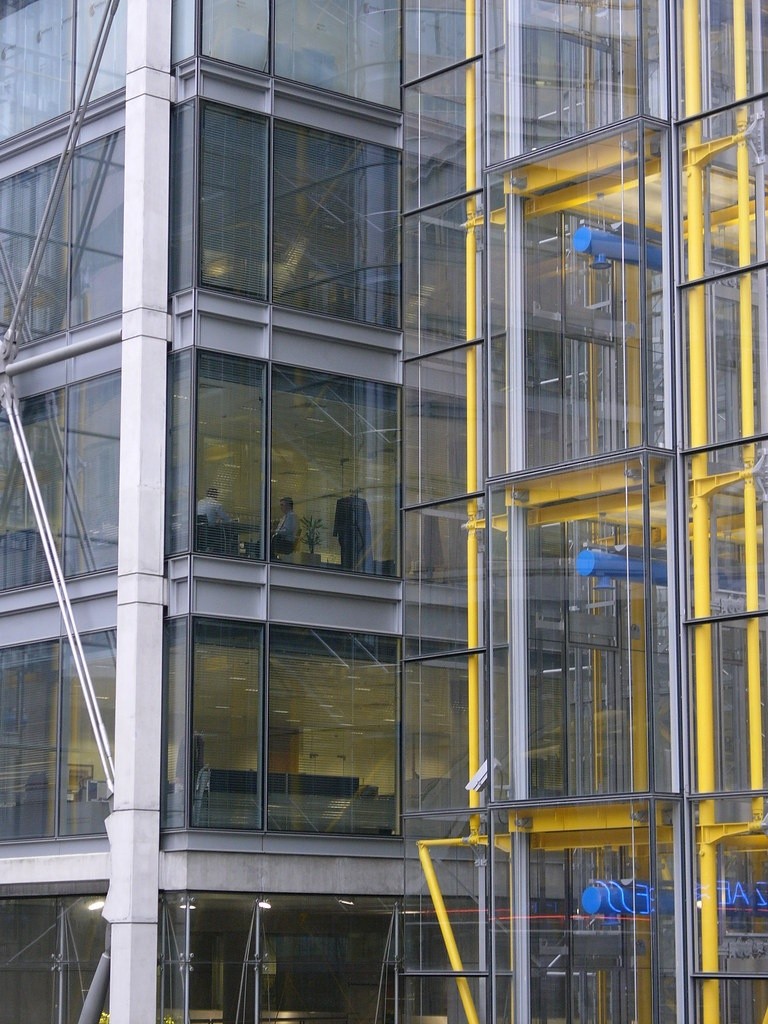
<box><xmin>271</xmin><ymin>529</ymin><xmax>302</xmax><ymax>560</ymax></box>
<box><xmin>196</xmin><ymin>513</ymin><xmax>226</xmax><ymax>554</ymax></box>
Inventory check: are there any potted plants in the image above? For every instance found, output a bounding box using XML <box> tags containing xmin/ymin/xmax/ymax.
<box><xmin>298</xmin><ymin>515</ymin><xmax>323</xmax><ymax>565</ymax></box>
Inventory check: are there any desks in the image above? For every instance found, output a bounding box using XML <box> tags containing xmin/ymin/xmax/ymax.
<box><xmin>219</xmin><ymin>522</ymin><xmax>260</xmax><ymax>555</ymax></box>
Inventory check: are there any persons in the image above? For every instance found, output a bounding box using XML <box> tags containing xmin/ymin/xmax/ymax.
<box><xmin>254</xmin><ymin>497</ymin><xmax>298</xmax><ymax>559</ymax></box>
<box><xmin>197</xmin><ymin>488</ymin><xmax>235</xmax><ymax>526</ymax></box>
<box><xmin>333</xmin><ymin>487</ymin><xmax>370</xmax><ymax>566</ymax></box>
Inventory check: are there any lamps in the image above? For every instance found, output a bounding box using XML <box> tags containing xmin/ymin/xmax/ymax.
<box><xmin>624</xmin><ymin>468</ymin><xmax>641</xmax><ymax>481</ymax></box>
<box><xmin>510</xmin><ymin>490</ymin><xmax>529</xmax><ymax>502</ymax></box>
<box><xmin>179</xmin><ymin>894</ymin><xmax>198</xmax><ymax>910</ymax></box>
<box><xmin>255</xmin><ymin>896</ymin><xmax>272</xmax><ymax>909</ymax></box>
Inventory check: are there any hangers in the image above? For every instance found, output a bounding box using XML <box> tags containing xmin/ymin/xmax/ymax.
<box><xmin>349</xmin><ymin>489</ymin><xmax>354</xmax><ymax>496</ymax></box>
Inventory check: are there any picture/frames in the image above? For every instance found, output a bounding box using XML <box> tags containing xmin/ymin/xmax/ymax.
<box><xmin>67</xmin><ymin>763</ymin><xmax>93</xmax><ymax>802</ymax></box>
